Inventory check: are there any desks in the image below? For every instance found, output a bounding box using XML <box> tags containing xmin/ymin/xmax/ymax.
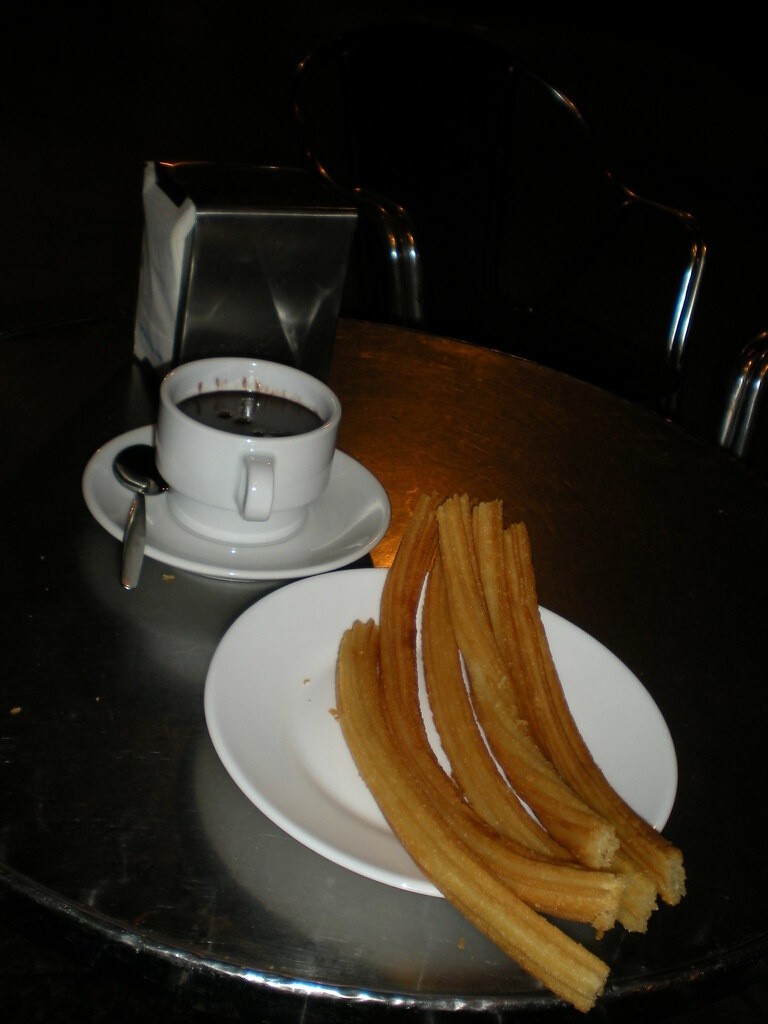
<box><xmin>0</xmin><ymin>320</ymin><xmax>768</xmax><ymax>1024</ymax></box>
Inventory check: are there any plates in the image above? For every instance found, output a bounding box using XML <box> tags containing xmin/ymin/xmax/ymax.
<box><xmin>80</xmin><ymin>422</ymin><xmax>390</xmax><ymax>583</ymax></box>
<box><xmin>202</xmin><ymin>568</ymin><xmax>678</xmax><ymax>899</ymax></box>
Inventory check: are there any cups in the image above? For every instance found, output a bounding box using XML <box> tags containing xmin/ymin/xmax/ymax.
<box><xmin>154</xmin><ymin>357</ymin><xmax>342</xmax><ymax>509</ymax></box>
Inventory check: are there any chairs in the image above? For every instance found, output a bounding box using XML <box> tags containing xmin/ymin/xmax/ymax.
<box><xmin>293</xmin><ymin>16</ymin><xmax>703</xmax><ymax>418</ymax></box>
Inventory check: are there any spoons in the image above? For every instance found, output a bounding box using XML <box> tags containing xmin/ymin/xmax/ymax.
<box><xmin>113</xmin><ymin>444</ymin><xmax>171</xmax><ymax>590</ymax></box>
